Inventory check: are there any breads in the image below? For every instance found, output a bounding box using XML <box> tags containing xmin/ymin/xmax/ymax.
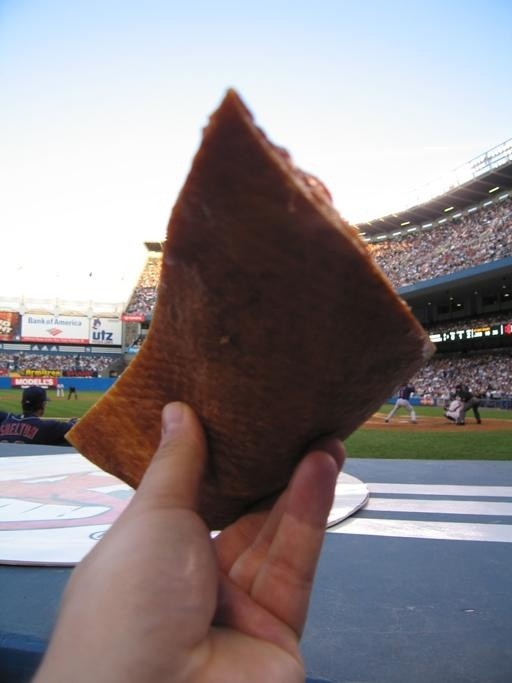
<box><xmin>64</xmin><ymin>87</ymin><xmax>437</xmax><ymax>533</ymax></box>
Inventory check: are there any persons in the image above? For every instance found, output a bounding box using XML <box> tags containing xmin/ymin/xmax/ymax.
<box><xmin>26</xmin><ymin>399</ymin><xmax>348</xmax><ymax>681</ymax></box>
<box><xmin>407</xmin><ymin>352</ymin><xmax>510</xmax><ymax>399</ymax></box>
<box><xmin>67</xmin><ymin>385</ymin><xmax>78</xmax><ymax>400</ymax></box>
<box><xmin>443</xmin><ymin>392</ymin><xmax>463</xmax><ymax>423</ymax></box>
<box><xmin>1</xmin><ymin>351</ymin><xmax>117</xmax><ymax>378</ymax></box>
<box><xmin>1</xmin><ymin>382</ymin><xmax>79</xmax><ymax>447</ymax></box>
<box><xmin>126</xmin><ymin>285</ymin><xmax>157</xmax><ymax>313</ymax></box>
<box><xmin>133</xmin><ymin>335</ymin><xmax>146</xmax><ymax>348</ymax></box>
<box><xmin>422</xmin><ymin>308</ymin><xmax>510</xmax><ymax>336</ymax></box>
<box><xmin>57</xmin><ymin>381</ymin><xmax>65</xmax><ymax>398</ymax></box>
<box><xmin>452</xmin><ymin>383</ymin><xmax>483</xmax><ymax>425</ymax></box>
<box><xmin>385</xmin><ymin>382</ymin><xmax>418</xmax><ymax>424</ymax></box>
<box><xmin>366</xmin><ymin>198</ymin><xmax>510</xmax><ymax>289</ymax></box>
